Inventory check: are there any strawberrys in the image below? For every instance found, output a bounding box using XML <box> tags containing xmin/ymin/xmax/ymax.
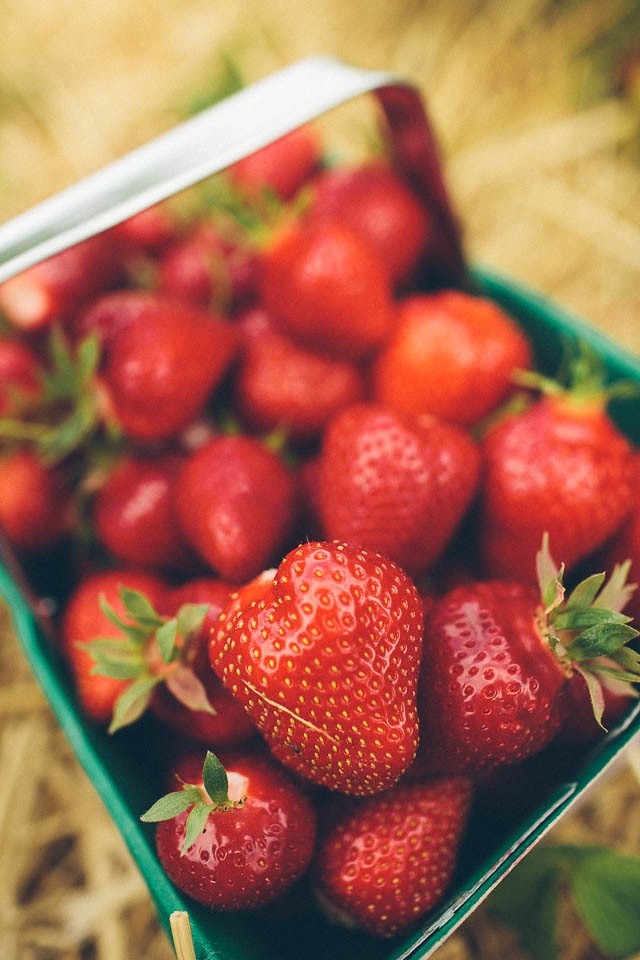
<box><xmin>0</xmin><ymin>126</ymin><xmax>329</xmax><ymax>444</ymax></box>
<box><xmin>0</xmin><ymin>166</ymin><xmax>640</xmax><ymax>939</ymax></box>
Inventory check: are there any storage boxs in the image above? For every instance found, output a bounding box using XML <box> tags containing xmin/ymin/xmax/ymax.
<box><xmin>0</xmin><ymin>61</ymin><xmax>640</xmax><ymax>960</ymax></box>
